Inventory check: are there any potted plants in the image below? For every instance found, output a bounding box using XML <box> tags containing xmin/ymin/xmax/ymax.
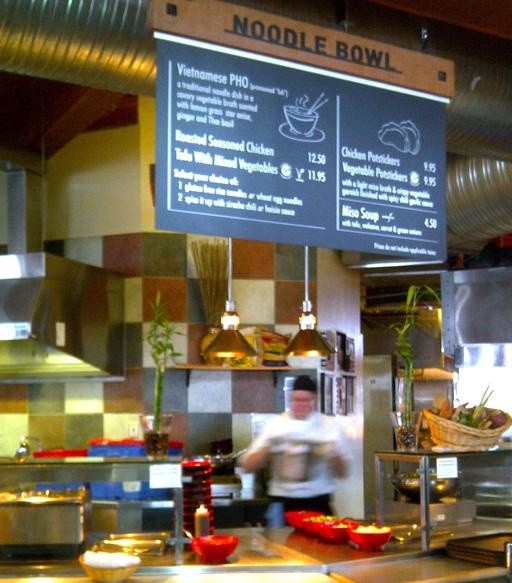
<box><xmin>388</xmin><ymin>284</ymin><xmax>442</xmax><ymax>451</ymax></box>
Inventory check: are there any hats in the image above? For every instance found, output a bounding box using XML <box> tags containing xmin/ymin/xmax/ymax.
<box><xmin>294</xmin><ymin>375</ymin><xmax>314</xmax><ymax>390</ymax></box>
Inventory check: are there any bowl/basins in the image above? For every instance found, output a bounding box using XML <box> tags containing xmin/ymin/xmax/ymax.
<box><xmin>181</xmin><ymin>459</ymin><xmax>217</xmax><ymax>536</ymax></box>
<box><xmin>80</xmin><ymin>551</ymin><xmax>141</xmax><ymax>582</ymax></box>
<box><xmin>284</xmin><ymin>509</ymin><xmax>391</xmax><ymax>550</ymax></box>
<box><xmin>189</xmin><ymin>533</ymin><xmax>239</xmax><ymax>561</ymax></box>
<box><xmin>203</xmin><ymin>447</ymin><xmax>247</xmax><ymax>475</ymax></box>
<box><xmin>389</xmin><ymin>472</ymin><xmax>461</xmax><ymax>500</ymax></box>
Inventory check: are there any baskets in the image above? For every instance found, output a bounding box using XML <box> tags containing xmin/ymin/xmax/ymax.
<box><xmin>422</xmin><ymin>407</ymin><xmax>512</xmax><ymax>451</ymax></box>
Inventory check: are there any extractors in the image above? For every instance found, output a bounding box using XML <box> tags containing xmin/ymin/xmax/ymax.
<box><xmin>0</xmin><ymin>140</ymin><xmax>126</xmax><ymax>381</ymax></box>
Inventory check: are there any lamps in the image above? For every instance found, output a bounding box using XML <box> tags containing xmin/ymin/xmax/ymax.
<box><xmin>202</xmin><ymin>237</ymin><xmax>334</xmax><ymax>359</ymax></box>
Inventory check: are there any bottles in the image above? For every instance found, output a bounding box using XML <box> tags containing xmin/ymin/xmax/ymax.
<box><xmin>503</xmin><ymin>542</ymin><xmax>511</xmax><ymax>568</ymax></box>
<box><xmin>194</xmin><ymin>505</ymin><xmax>211</xmax><ymax>538</ymax></box>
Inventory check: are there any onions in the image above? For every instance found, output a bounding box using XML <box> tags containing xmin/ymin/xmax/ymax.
<box><xmin>488</xmin><ymin>414</ymin><xmax>506</xmax><ymax>428</ymax></box>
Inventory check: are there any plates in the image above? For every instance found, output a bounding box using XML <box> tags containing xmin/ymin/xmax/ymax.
<box><xmin>34</xmin><ymin>438</ymin><xmax>185</xmax><ymax>458</ymax></box>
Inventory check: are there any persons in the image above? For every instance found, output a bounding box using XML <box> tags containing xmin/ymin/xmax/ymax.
<box><xmin>235</xmin><ymin>374</ymin><xmax>351</xmax><ymax>528</ymax></box>
